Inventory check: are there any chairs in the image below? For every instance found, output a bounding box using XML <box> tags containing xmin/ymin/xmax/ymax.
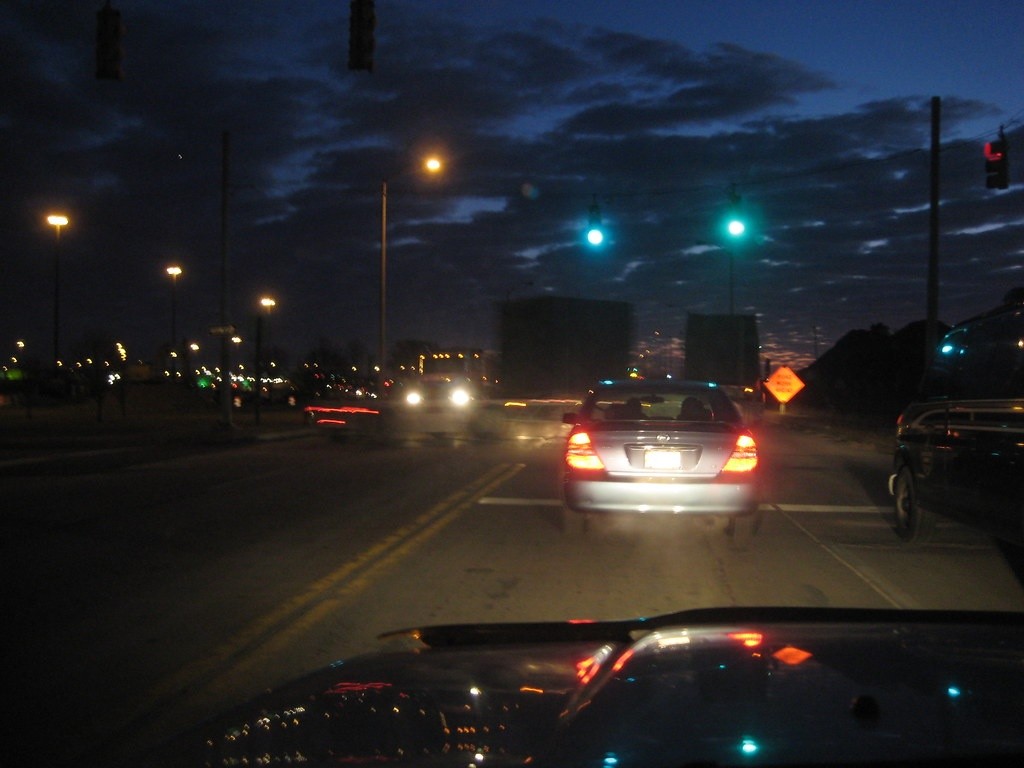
<box><xmin>675</xmin><ymin>406</ymin><xmax>712</xmax><ymax>421</ymax></box>
<box><xmin>604</xmin><ymin>401</ymin><xmax>643</xmax><ymax>419</ymax></box>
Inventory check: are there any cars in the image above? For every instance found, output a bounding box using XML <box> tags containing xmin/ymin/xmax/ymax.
<box><xmin>400</xmin><ymin>372</ymin><xmax>475</xmax><ymax>420</ymax></box>
<box><xmin>563</xmin><ymin>376</ymin><xmax>764</xmax><ymax>528</ymax></box>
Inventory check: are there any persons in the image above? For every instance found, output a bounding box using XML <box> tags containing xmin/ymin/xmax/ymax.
<box><xmin>627</xmin><ymin>397</ymin><xmax>649</xmax><ymax>420</ymax></box>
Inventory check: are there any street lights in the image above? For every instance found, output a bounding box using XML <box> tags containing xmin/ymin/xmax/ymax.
<box><xmin>45</xmin><ymin>213</ymin><xmax>70</xmax><ymax>357</ymax></box>
<box><xmin>259</xmin><ymin>295</ymin><xmax>276</xmax><ymax>395</ymax></box>
<box><xmin>167</xmin><ymin>264</ymin><xmax>183</xmax><ymax>372</ymax></box>
<box><xmin>377</xmin><ymin>148</ymin><xmax>445</xmax><ymax>400</ymax></box>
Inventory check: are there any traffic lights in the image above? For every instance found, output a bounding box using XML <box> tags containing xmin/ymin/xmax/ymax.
<box><xmin>984</xmin><ymin>140</ymin><xmax>1009</xmax><ymax>189</ymax></box>
<box><xmin>726</xmin><ymin>202</ymin><xmax>747</xmax><ymax>243</ymax></box>
<box><xmin>587</xmin><ymin>207</ymin><xmax>604</xmax><ymax>248</ymax></box>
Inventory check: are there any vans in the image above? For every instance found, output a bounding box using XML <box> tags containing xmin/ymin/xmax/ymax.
<box><xmin>892</xmin><ymin>308</ymin><xmax>1023</xmax><ymax>551</ymax></box>
